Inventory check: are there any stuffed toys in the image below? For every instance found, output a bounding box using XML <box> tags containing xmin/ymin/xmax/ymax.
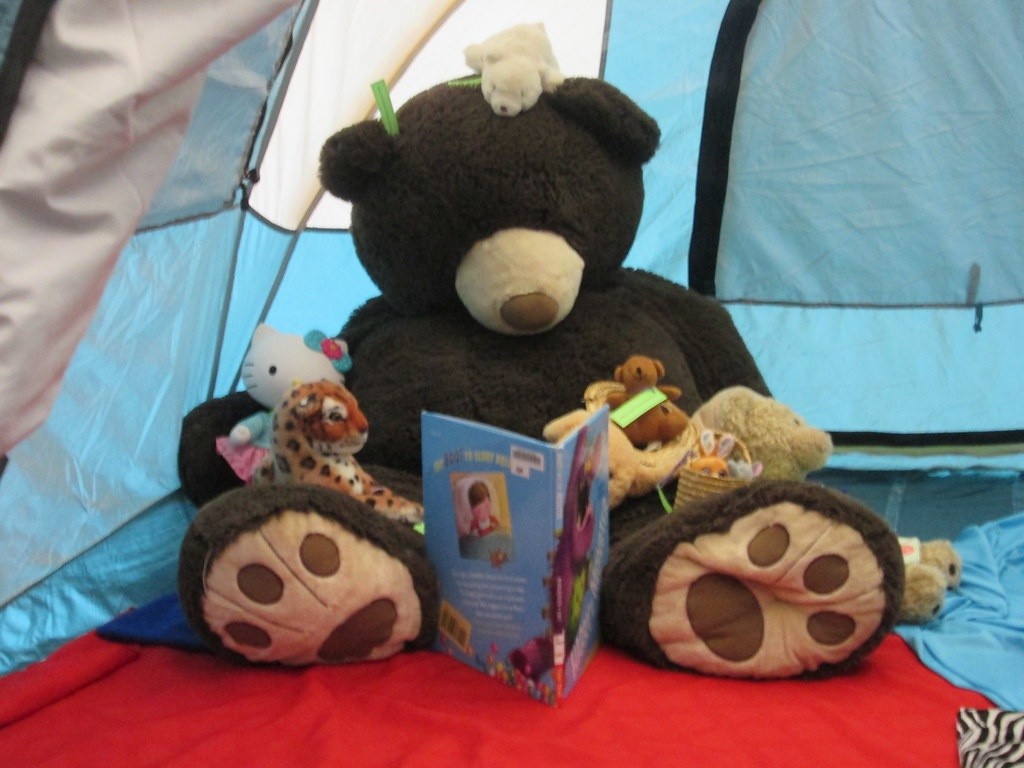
<box><xmin>177</xmin><ymin>25</ymin><xmax>961</xmax><ymax>679</ymax></box>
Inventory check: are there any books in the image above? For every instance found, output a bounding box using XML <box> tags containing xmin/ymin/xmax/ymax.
<box><xmin>421</xmin><ymin>406</ymin><xmax>609</xmax><ymax>709</ymax></box>
